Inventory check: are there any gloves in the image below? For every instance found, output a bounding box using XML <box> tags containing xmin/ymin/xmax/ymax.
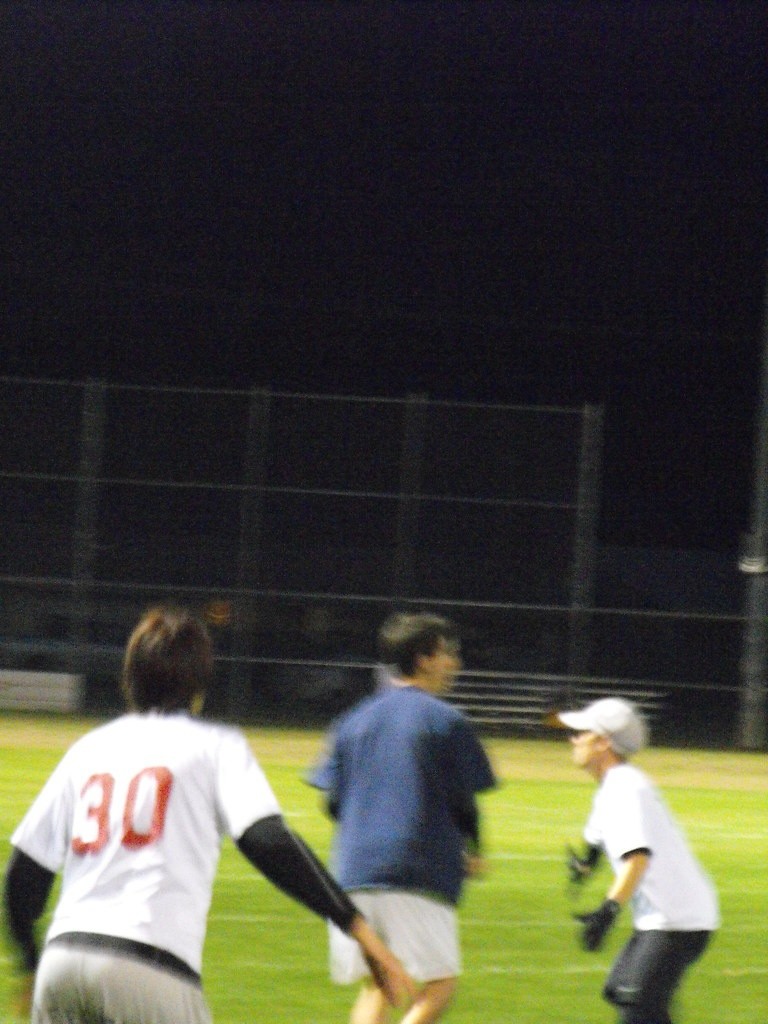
<box><xmin>572</xmin><ymin>848</ymin><xmax>601</xmax><ymax>881</ymax></box>
<box><xmin>572</xmin><ymin>900</ymin><xmax>620</xmax><ymax>952</ymax></box>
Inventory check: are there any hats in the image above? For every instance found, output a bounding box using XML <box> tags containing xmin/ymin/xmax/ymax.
<box><xmin>559</xmin><ymin>697</ymin><xmax>643</xmax><ymax>759</ymax></box>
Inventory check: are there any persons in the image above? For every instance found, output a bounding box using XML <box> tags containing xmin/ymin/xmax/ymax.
<box><xmin>558</xmin><ymin>698</ymin><xmax>720</xmax><ymax>1024</ymax></box>
<box><xmin>299</xmin><ymin>612</ymin><xmax>499</xmax><ymax>1024</ymax></box>
<box><xmin>7</xmin><ymin>605</ymin><xmax>418</xmax><ymax>1024</ymax></box>
